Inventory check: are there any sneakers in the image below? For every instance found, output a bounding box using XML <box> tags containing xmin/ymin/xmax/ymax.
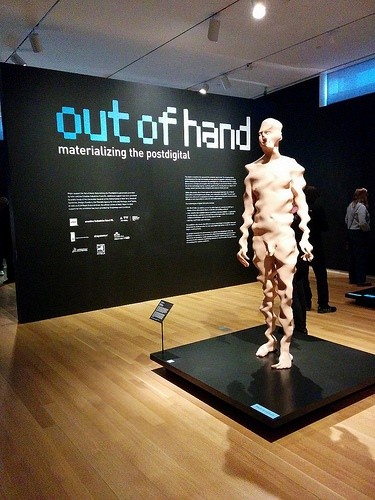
<box><xmin>306</xmin><ymin>303</ymin><xmax>311</xmax><ymax>311</ymax></box>
<box><xmin>318</xmin><ymin>306</ymin><xmax>336</xmax><ymax>313</ymax></box>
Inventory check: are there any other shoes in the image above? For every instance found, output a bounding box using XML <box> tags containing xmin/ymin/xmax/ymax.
<box><xmin>350</xmin><ymin>282</ymin><xmax>356</xmax><ymax>283</ymax></box>
<box><xmin>357</xmin><ymin>282</ymin><xmax>372</xmax><ymax>286</ymax></box>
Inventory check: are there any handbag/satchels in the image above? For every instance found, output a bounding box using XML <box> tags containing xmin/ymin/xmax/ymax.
<box><xmin>346</xmin><ymin>230</ymin><xmax>352</xmax><ymax>241</ymax></box>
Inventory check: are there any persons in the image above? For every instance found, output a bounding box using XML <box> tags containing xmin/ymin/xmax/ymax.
<box><xmin>236</xmin><ymin>117</ymin><xmax>315</xmax><ymax>370</ymax></box>
<box><xmin>345</xmin><ymin>187</ymin><xmax>372</xmax><ymax>286</ymax></box>
<box><xmin>290</xmin><ymin>184</ymin><xmax>337</xmax><ymax>313</ymax></box>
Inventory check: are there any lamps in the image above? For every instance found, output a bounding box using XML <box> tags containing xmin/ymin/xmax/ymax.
<box><xmin>207</xmin><ymin>17</ymin><xmax>220</xmax><ymax>42</ymax></box>
<box><xmin>11</xmin><ymin>54</ymin><xmax>27</xmax><ymax>66</ymax></box>
<box><xmin>199</xmin><ymin>84</ymin><xmax>209</xmax><ymax>94</ymax></box>
<box><xmin>29</xmin><ymin>29</ymin><xmax>42</xmax><ymax>53</ymax></box>
<box><xmin>221</xmin><ymin>75</ymin><xmax>231</xmax><ymax>91</ymax></box>
